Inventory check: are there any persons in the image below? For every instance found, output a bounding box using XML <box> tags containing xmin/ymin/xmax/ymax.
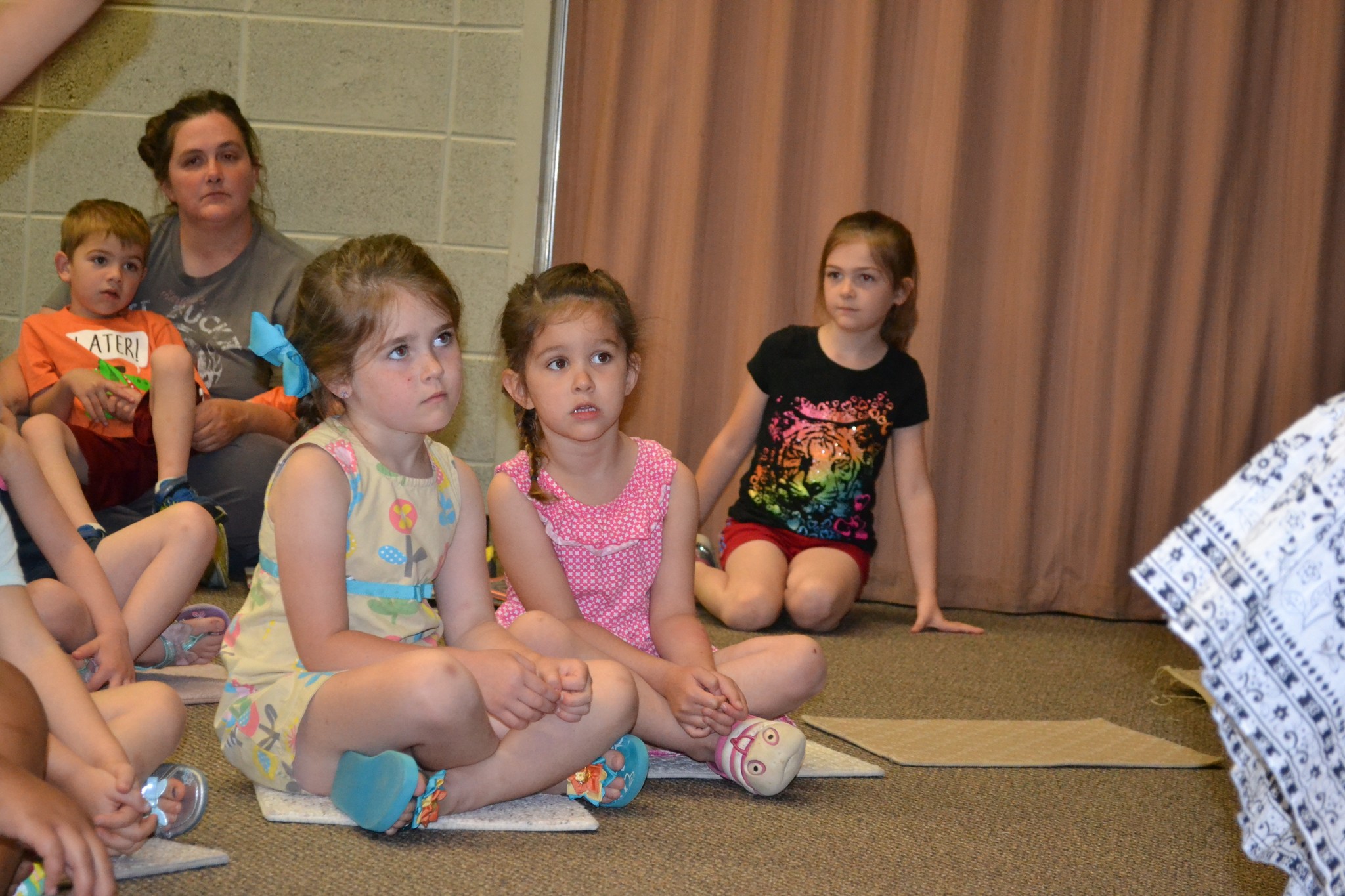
<box><xmin>0</xmin><ymin>658</ymin><xmax>120</xmax><ymax>895</ymax></box>
<box><xmin>0</xmin><ymin>92</ymin><xmax>320</xmax><ymax>584</ymax></box>
<box><xmin>212</xmin><ymin>235</ymin><xmax>650</xmax><ymax>835</ymax></box>
<box><xmin>484</xmin><ymin>260</ymin><xmax>822</xmax><ymax>799</ymax></box>
<box><xmin>1</xmin><ymin>418</ymin><xmax>230</xmax><ymax>690</ymax></box>
<box><xmin>0</xmin><ymin>499</ymin><xmax>210</xmax><ymax>856</ymax></box>
<box><xmin>23</xmin><ymin>198</ymin><xmax>299</xmax><ymax>555</ymax></box>
<box><xmin>689</xmin><ymin>211</ymin><xmax>986</xmax><ymax>635</ymax></box>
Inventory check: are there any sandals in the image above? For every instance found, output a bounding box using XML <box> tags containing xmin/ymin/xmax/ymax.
<box><xmin>133</xmin><ymin>603</ymin><xmax>230</xmax><ymax>672</ymax></box>
<box><xmin>141</xmin><ymin>762</ymin><xmax>207</xmax><ymax>843</ymax></box>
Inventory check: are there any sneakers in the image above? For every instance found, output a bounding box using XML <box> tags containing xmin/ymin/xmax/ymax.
<box><xmin>152</xmin><ymin>474</ymin><xmax>229</xmax><ymax>525</ymax></box>
<box><xmin>74</xmin><ymin>524</ymin><xmax>106</xmax><ymax>556</ymax></box>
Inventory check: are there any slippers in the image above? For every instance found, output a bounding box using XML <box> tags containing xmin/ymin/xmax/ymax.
<box><xmin>707</xmin><ymin>714</ymin><xmax>806</xmax><ymax>797</ymax></box>
<box><xmin>328</xmin><ymin>749</ymin><xmax>448</xmax><ymax>834</ymax></box>
<box><xmin>566</xmin><ymin>734</ymin><xmax>650</xmax><ymax>809</ymax></box>
